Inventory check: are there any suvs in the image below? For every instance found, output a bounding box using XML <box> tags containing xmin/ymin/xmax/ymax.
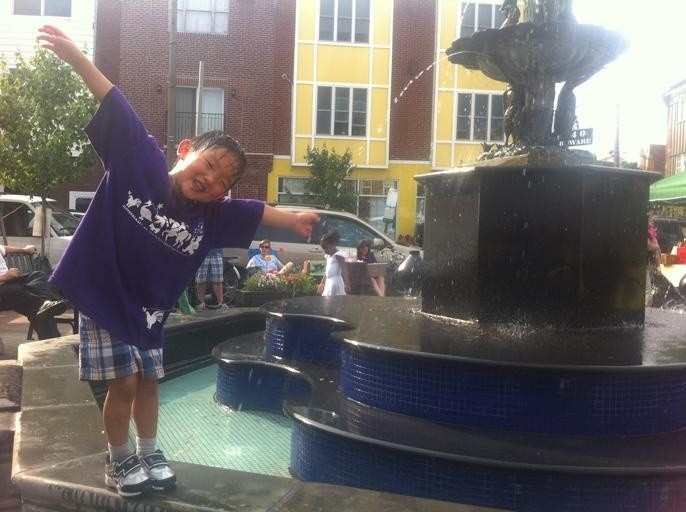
<box><xmin>221</xmin><ymin>205</ymin><xmax>422</xmax><ymax>289</ymax></box>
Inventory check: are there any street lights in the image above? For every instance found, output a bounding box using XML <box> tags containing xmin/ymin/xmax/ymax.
<box><xmin>380</xmin><ymin>180</ymin><xmax>399</xmax><ymax>233</ymax></box>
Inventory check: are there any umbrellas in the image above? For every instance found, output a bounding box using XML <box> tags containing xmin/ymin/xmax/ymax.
<box><xmin>649</xmin><ymin>173</ymin><xmax>686</xmax><ymax>201</ymax></box>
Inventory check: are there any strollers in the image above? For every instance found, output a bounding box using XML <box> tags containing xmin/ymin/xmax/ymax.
<box><xmin>652</xmin><ymin>269</ymin><xmax>686</xmax><ymax>314</ymax></box>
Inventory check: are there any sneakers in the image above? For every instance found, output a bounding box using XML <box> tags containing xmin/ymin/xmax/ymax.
<box><xmin>105</xmin><ymin>449</ymin><xmax>179</xmax><ymax>496</ymax></box>
<box><xmin>194</xmin><ymin>303</ymin><xmax>206</xmax><ymax>312</ymax></box>
<box><xmin>217</xmin><ymin>304</ymin><xmax>226</xmax><ymax>311</ymax></box>
<box><xmin>36</xmin><ymin>301</ymin><xmax>66</xmax><ymax>319</ymax></box>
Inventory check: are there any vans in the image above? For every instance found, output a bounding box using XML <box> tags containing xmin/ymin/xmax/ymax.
<box><xmin>0</xmin><ymin>194</ymin><xmax>82</xmax><ymax>270</ymax></box>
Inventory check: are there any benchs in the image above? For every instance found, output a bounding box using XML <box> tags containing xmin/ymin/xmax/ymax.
<box><xmin>0</xmin><ymin>249</ymin><xmax>78</xmax><ymax>341</ymax></box>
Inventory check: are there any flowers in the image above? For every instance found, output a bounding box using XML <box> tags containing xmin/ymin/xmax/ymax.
<box><xmin>240</xmin><ymin>272</ymin><xmax>317</xmax><ymax>291</ymax></box>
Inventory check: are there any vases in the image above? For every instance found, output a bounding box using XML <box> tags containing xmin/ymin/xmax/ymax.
<box><xmin>235</xmin><ymin>289</ymin><xmax>316</xmax><ymax>308</ymax></box>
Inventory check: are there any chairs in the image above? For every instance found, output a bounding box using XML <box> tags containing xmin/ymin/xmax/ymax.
<box><xmin>242</xmin><ymin>243</ymin><xmax>285</xmax><ymax>279</ymax></box>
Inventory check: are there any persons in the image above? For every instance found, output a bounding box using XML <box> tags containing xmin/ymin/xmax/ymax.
<box><xmin>37</xmin><ymin>21</ymin><xmax>321</xmax><ymax>499</ymax></box>
<box><xmin>357</xmin><ymin>238</ymin><xmax>385</xmax><ymax>297</ymax></box>
<box><xmin>398</xmin><ymin>232</ymin><xmax>423</xmax><ymax>249</ymax></box>
<box><xmin>321</xmin><ymin>231</ymin><xmax>351</xmax><ymax>296</ymax></box>
<box><xmin>0</xmin><ymin>243</ymin><xmax>70</xmax><ymax>340</ymax></box>
<box><xmin>246</xmin><ymin>239</ymin><xmax>293</xmax><ymax>277</ymax></box>
<box><xmin>196</xmin><ymin>247</ymin><xmax>228</xmax><ymax>311</ymax></box>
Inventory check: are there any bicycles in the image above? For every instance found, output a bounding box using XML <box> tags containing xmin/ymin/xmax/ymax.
<box><xmin>187</xmin><ymin>257</ymin><xmax>241</xmax><ymax>309</ymax></box>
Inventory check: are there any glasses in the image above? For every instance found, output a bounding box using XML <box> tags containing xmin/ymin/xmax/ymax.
<box><xmin>260</xmin><ymin>244</ymin><xmax>270</xmax><ymax>249</ymax></box>
